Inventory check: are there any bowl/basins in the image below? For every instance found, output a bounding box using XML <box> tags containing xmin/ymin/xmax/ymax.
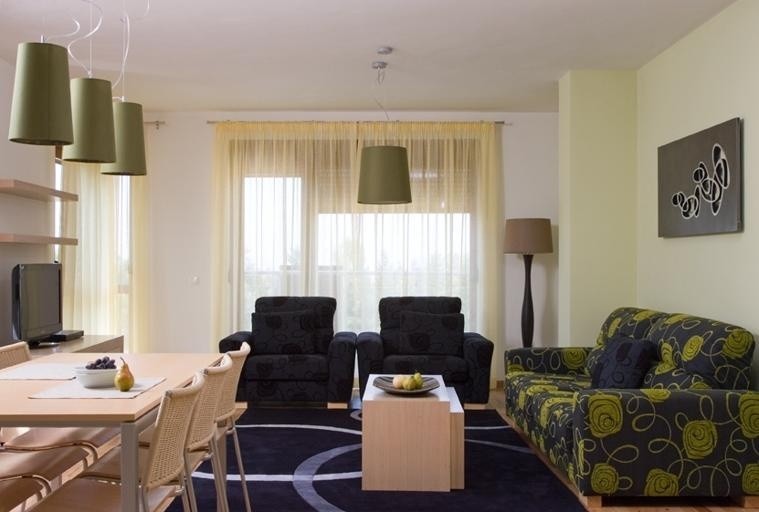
<box><xmin>71</xmin><ymin>355</ymin><xmax>121</xmax><ymax>389</ymax></box>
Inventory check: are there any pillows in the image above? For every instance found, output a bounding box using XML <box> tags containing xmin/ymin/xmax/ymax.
<box><xmin>590</xmin><ymin>332</ymin><xmax>660</xmax><ymax>390</ymax></box>
<box><xmin>398</xmin><ymin>310</ymin><xmax>465</xmax><ymax>356</ymax></box>
<box><xmin>251</xmin><ymin>310</ymin><xmax>317</xmax><ymax>355</ymax></box>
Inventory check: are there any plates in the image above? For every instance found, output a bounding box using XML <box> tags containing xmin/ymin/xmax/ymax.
<box><xmin>370</xmin><ymin>372</ymin><xmax>441</xmax><ymax>396</ymax></box>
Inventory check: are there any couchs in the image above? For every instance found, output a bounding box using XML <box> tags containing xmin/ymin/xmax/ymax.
<box><xmin>219</xmin><ymin>296</ymin><xmax>358</xmax><ymax>410</ymax></box>
<box><xmin>356</xmin><ymin>297</ymin><xmax>494</xmax><ymax>405</ymax></box>
<box><xmin>504</xmin><ymin>306</ymin><xmax>759</xmax><ymax>508</ymax></box>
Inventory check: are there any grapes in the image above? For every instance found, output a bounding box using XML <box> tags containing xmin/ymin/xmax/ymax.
<box><xmin>83</xmin><ymin>355</ymin><xmax>115</xmax><ymax>371</ymax></box>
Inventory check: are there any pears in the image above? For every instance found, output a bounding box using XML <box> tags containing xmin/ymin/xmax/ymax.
<box><xmin>114</xmin><ymin>357</ymin><xmax>134</xmax><ymax>391</ymax></box>
<box><xmin>393</xmin><ymin>375</ymin><xmax>404</xmax><ymax>389</ymax></box>
<box><xmin>413</xmin><ymin>372</ymin><xmax>423</xmax><ymax>388</ymax></box>
<box><xmin>403</xmin><ymin>376</ymin><xmax>416</xmax><ymax>391</ymax></box>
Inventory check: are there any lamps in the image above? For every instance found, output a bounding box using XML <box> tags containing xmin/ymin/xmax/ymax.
<box><xmin>60</xmin><ymin>3</ymin><xmax>117</xmax><ymax>164</ymax></box>
<box><xmin>503</xmin><ymin>217</ymin><xmax>554</xmax><ymax>349</ymax></box>
<box><xmin>7</xmin><ymin>8</ymin><xmax>75</xmax><ymax>146</ymax></box>
<box><xmin>100</xmin><ymin>0</ymin><xmax>148</xmax><ymax>176</ymax></box>
<box><xmin>356</xmin><ymin>61</ymin><xmax>413</xmax><ymax>205</ymax></box>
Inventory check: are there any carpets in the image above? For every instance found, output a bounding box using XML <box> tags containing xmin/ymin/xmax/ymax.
<box><xmin>164</xmin><ymin>409</ymin><xmax>588</xmax><ymax>512</ymax></box>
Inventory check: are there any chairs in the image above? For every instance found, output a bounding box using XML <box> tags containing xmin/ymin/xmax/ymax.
<box><xmin>0</xmin><ymin>445</ymin><xmax>92</xmax><ymax>496</ymax></box>
<box><xmin>0</xmin><ymin>341</ymin><xmax>122</xmax><ymax>463</ymax></box>
<box><xmin>118</xmin><ymin>340</ymin><xmax>252</xmax><ymax>512</ymax></box>
<box><xmin>73</xmin><ymin>354</ymin><xmax>233</xmax><ymax>511</ymax></box>
<box><xmin>0</xmin><ymin>476</ymin><xmax>43</xmax><ymax>512</ymax></box>
<box><xmin>24</xmin><ymin>370</ymin><xmax>206</xmax><ymax>512</ymax></box>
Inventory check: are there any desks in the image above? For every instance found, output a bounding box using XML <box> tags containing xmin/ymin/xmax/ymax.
<box><xmin>28</xmin><ymin>333</ymin><xmax>125</xmax><ymax>360</ymax></box>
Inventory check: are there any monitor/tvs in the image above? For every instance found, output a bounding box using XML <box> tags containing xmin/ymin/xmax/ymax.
<box><xmin>11</xmin><ymin>263</ymin><xmax>64</xmax><ymax>349</ymax></box>
